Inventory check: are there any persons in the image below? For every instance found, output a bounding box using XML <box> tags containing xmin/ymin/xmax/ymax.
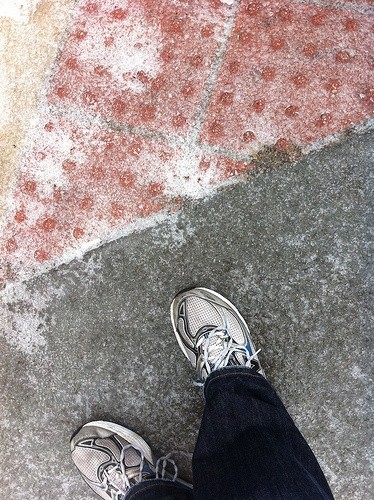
<box><xmin>69</xmin><ymin>284</ymin><xmax>337</xmax><ymax>500</ymax></box>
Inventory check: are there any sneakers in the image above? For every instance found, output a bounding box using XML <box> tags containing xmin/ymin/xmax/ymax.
<box><xmin>169</xmin><ymin>286</ymin><xmax>268</xmax><ymax>404</ymax></box>
<box><xmin>69</xmin><ymin>420</ymin><xmax>195</xmax><ymax>500</ymax></box>
